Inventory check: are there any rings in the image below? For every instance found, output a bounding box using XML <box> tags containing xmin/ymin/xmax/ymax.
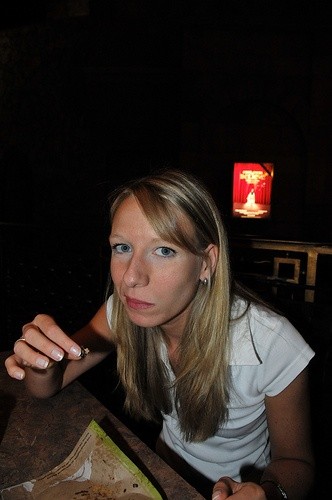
<box><xmin>14</xmin><ymin>338</ymin><xmax>25</xmax><ymax>346</ymax></box>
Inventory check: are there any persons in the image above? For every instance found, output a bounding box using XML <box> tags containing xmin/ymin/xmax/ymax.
<box><xmin>3</xmin><ymin>165</ymin><xmax>316</xmax><ymax>500</ymax></box>
<box><xmin>246</xmin><ymin>188</ymin><xmax>255</xmax><ymax>212</ymax></box>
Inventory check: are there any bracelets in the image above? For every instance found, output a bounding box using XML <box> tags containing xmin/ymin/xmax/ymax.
<box><xmin>259</xmin><ymin>478</ymin><xmax>288</xmax><ymax>500</ymax></box>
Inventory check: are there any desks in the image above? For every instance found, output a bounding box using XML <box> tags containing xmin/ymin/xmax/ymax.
<box><xmin>0</xmin><ymin>325</ymin><xmax>233</xmax><ymax>500</ymax></box>
<box><xmin>233</xmin><ymin>238</ymin><xmax>332</xmax><ymax>304</ymax></box>
